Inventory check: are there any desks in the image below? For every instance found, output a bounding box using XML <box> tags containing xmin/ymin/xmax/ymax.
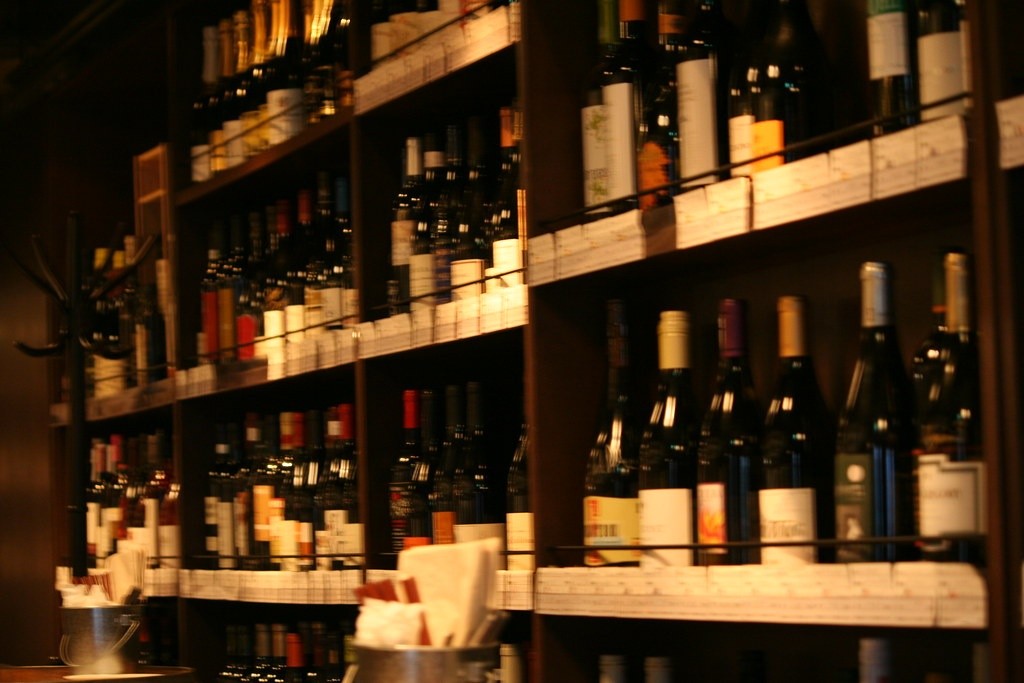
<box><xmin>0</xmin><ymin>665</ymin><xmax>196</xmax><ymax>683</ymax></box>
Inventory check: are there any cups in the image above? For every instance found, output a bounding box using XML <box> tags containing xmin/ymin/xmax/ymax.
<box><xmin>349</xmin><ymin>639</ymin><xmax>501</xmax><ymax>683</ymax></box>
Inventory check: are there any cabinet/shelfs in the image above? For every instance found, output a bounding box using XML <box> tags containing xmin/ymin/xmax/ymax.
<box><xmin>86</xmin><ymin>0</ymin><xmax>1024</xmax><ymax>683</ymax></box>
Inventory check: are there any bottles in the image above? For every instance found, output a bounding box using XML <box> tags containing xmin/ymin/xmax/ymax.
<box><xmin>566</xmin><ymin>0</ymin><xmax>972</xmax><ymax>221</ymax></box>
<box><xmin>499</xmin><ymin>646</ymin><xmax>523</xmax><ymax>683</ymax></box>
<box><xmin>581</xmin><ymin>254</ymin><xmax>988</xmax><ymax>566</ymax></box>
<box><xmin>645</xmin><ymin>656</ymin><xmax>670</xmax><ymax>683</ymax></box>
<box><xmin>212</xmin><ymin>621</ymin><xmax>358</xmax><ymax>683</ymax></box>
<box><xmin>971</xmin><ymin>643</ymin><xmax>990</xmax><ymax>683</ymax></box>
<box><xmin>88</xmin><ymin>184</ymin><xmax>354</xmax><ymax>394</ymax></box>
<box><xmin>193</xmin><ymin>0</ymin><xmax>360</xmax><ymax>178</ymax></box>
<box><xmin>394</xmin><ymin>107</ymin><xmax>522</xmax><ymax>312</ymax></box>
<box><xmin>381</xmin><ymin>390</ymin><xmax>530</xmax><ymax>546</ymax></box>
<box><xmin>858</xmin><ymin>636</ymin><xmax>889</xmax><ymax>683</ymax></box>
<box><xmin>600</xmin><ymin>656</ymin><xmax>623</xmax><ymax>683</ymax></box>
<box><xmin>85</xmin><ymin>407</ymin><xmax>360</xmax><ymax>570</ymax></box>
